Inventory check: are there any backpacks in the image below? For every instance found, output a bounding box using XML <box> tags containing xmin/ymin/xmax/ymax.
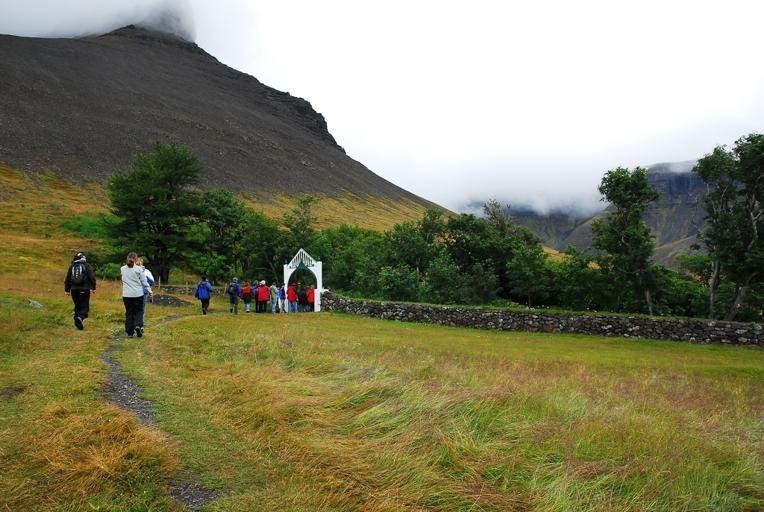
<box><xmin>70</xmin><ymin>263</ymin><xmax>87</xmax><ymax>284</ymax></box>
<box><xmin>228</xmin><ymin>283</ymin><xmax>236</xmax><ymax>294</ymax></box>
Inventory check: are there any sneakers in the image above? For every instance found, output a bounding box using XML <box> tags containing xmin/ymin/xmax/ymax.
<box><xmin>74</xmin><ymin>316</ymin><xmax>84</xmax><ymax>330</ymax></box>
<box><xmin>128</xmin><ymin>325</ymin><xmax>144</xmax><ymax>338</ymax></box>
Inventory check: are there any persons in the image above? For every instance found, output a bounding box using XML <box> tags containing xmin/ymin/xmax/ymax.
<box><xmin>65</xmin><ymin>252</ymin><xmax>96</xmax><ymax>330</ymax></box>
<box><xmin>195</xmin><ymin>276</ymin><xmax>213</xmax><ymax>315</ymax></box>
<box><xmin>121</xmin><ymin>252</ymin><xmax>154</xmax><ymax>337</ymax></box>
<box><xmin>228</xmin><ymin>277</ymin><xmax>314</xmax><ymax>314</ymax></box>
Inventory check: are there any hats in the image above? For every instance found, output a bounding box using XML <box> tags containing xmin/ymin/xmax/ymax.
<box><xmin>259</xmin><ymin>280</ymin><xmax>266</xmax><ymax>285</ymax></box>
